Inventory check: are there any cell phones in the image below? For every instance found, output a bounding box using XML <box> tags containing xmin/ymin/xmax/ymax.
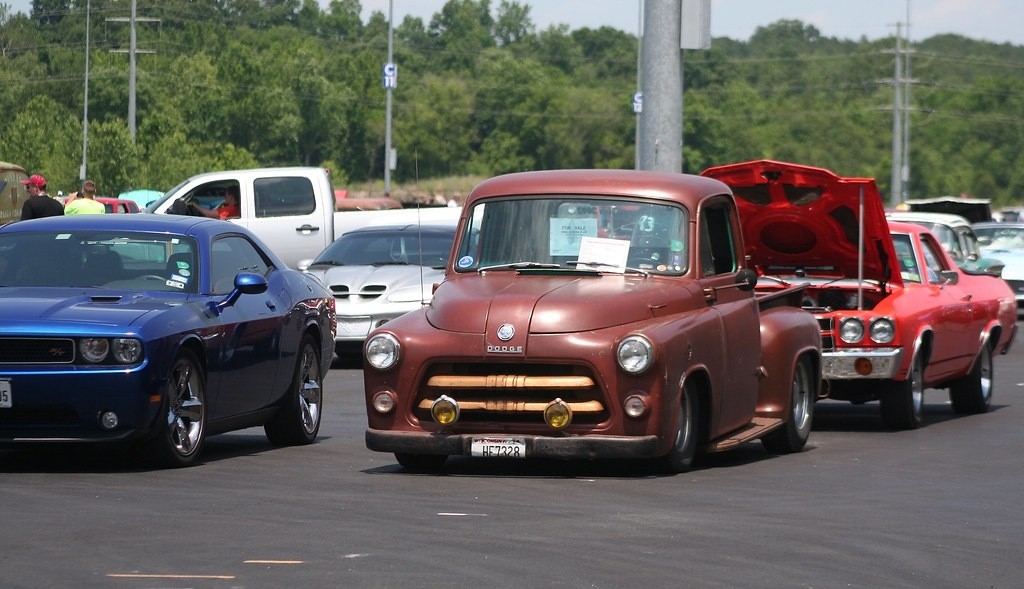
<box><xmin>77</xmin><ymin>192</ymin><xmax>84</xmax><ymax>196</ymax></box>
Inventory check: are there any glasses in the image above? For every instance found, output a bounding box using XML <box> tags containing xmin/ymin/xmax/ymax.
<box><xmin>27</xmin><ymin>186</ymin><xmax>29</xmax><ymax>189</ymax></box>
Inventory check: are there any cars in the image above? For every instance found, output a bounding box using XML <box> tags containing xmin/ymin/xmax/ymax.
<box><xmin>686</xmin><ymin>159</ymin><xmax>1024</xmax><ymax>430</ymax></box>
<box><xmin>0</xmin><ymin>214</ymin><xmax>338</xmax><ymax>464</ymax></box>
<box><xmin>0</xmin><ymin>162</ymin><xmax>162</xmax><ymax>230</ymax></box>
<box><xmin>310</xmin><ymin>169</ymin><xmax>824</xmax><ymax>475</ymax></box>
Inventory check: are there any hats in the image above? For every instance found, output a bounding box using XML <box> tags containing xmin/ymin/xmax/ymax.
<box><xmin>20</xmin><ymin>175</ymin><xmax>46</xmax><ymax>186</ymax></box>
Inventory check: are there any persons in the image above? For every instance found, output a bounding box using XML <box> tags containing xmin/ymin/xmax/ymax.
<box><xmin>187</xmin><ymin>186</ymin><xmax>241</xmax><ymax>219</ymax></box>
<box><xmin>63</xmin><ymin>181</ymin><xmax>105</xmax><ymax>215</ymax></box>
<box><xmin>20</xmin><ymin>174</ymin><xmax>63</xmax><ymax>221</ymax></box>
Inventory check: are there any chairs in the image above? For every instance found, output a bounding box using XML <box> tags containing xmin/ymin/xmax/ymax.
<box><xmin>163</xmin><ymin>253</ymin><xmax>215</xmax><ymax>293</ymax></box>
<box><xmin>76</xmin><ymin>250</ymin><xmax>125</xmax><ymax>285</ymax></box>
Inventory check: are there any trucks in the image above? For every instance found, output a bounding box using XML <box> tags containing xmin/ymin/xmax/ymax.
<box><xmin>114</xmin><ymin>165</ymin><xmax>488</xmax><ymax>274</ymax></box>
